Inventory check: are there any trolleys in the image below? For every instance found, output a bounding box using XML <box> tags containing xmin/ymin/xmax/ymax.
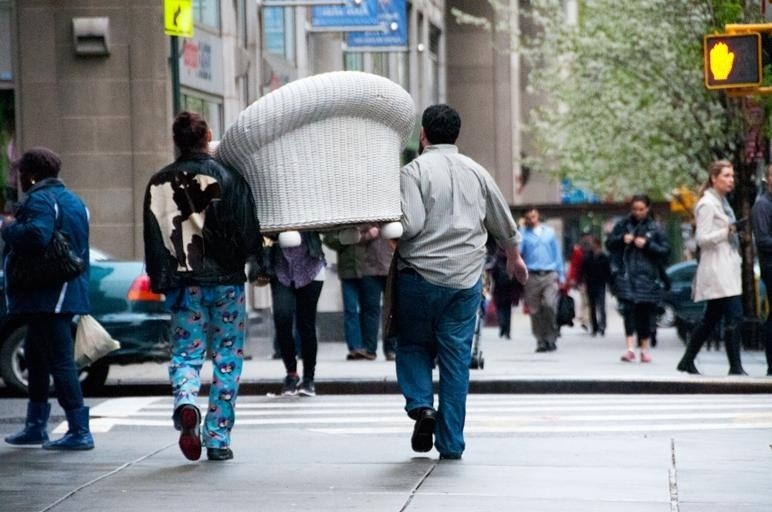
<box><xmin>382</xmin><ymin>254</ymin><xmax>490</xmax><ymax>369</ymax></box>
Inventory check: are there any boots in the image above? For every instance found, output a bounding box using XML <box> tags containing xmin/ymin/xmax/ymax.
<box><xmin>45</xmin><ymin>407</ymin><xmax>95</xmax><ymax>450</ymax></box>
<box><xmin>5</xmin><ymin>404</ymin><xmax>52</xmax><ymax>445</ymax></box>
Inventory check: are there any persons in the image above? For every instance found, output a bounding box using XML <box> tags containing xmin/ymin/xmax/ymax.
<box><xmin>144</xmin><ymin>110</ymin><xmax>264</xmax><ymax>462</ymax></box>
<box><xmin>397</xmin><ymin>104</ymin><xmax>529</xmax><ymax>461</ymax></box>
<box><xmin>0</xmin><ymin>147</ymin><xmax>95</xmax><ymax>452</ymax></box>
<box><xmin>249</xmin><ymin>220</ymin><xmax>397</xmax><ymax>399</ymax></box>
<box><xmin>677</xmin><ymin>160</ymin><xmax>772</xmax><ymax>374</ymax></box>
<box><xmin>605</xmin><ymin>191</ymin><xmax>672</xmax><ymax>365</ymax></box>
<box><xmin>488</xmin><ymin>210</ymin><xmax>614</xmax><ymax>352</ymax></box>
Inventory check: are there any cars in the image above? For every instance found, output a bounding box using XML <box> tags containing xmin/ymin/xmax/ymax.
<box><xmin>0</xmin><ymin>236</ymin><xmax>174</xmax><ymax>397</ymax></box>
<box><xmin>620</xmin><ymin>259</ymin><xmax>699</xmax><ymax>329</ymax></box>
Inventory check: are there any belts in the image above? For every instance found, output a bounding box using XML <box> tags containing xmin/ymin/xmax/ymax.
<box><xmin>529</xmin><ymin>270</ymin><xmax>552</xmax><ymax>277</ymax></box>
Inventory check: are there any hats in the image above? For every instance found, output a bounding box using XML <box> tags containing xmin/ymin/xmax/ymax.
<box><xmin>11</xmin><ymin>148</ymin><xmax>60</xmax><ymax>180</ymax></box>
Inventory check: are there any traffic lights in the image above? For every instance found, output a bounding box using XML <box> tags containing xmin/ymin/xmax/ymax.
<box><xmin>703</xmin><ymin>30</ymin><xmax>762</xmax><ymax>89</ymax></box>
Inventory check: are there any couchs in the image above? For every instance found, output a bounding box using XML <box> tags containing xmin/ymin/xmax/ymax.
<box><xmin>212</xmin><ymin>70</ymin><xmax>418</xmax><ymax>239</ymax></box>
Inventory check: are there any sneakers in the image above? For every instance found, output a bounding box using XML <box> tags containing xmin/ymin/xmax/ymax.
<box><xmin>281</xmin><ymin>348</ymin><xmax>375</xmax><ymax>396</ymax></box>
<box><xmin>207</xmin><ymin>446</ymin><xmax>232</xmax><ymax>460</ymax></box>
<box><xmin>538</xmin><ymin>326</ymin><xmax>746</xmax><ymax>375</ymax></box>
<box><xmin>410</xmin><ymin>409</ymin><xmax>436</xmax><ymax>451</ymax></box>
<box><xmin>179</xmin><ymin>405</ymin><xmax>201</xmax><ymax>460</ymax></box>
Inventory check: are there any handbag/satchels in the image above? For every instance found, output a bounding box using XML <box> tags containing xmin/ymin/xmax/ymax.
<box><xmin>558</xmin><ymin>291</ymin><xmax>574</xmax><ymax>326</ymax></box>
<box><xmin>8</xmin><ymin>229</ymin><xmax>83</xmax><ymax>300</ymax></box>
